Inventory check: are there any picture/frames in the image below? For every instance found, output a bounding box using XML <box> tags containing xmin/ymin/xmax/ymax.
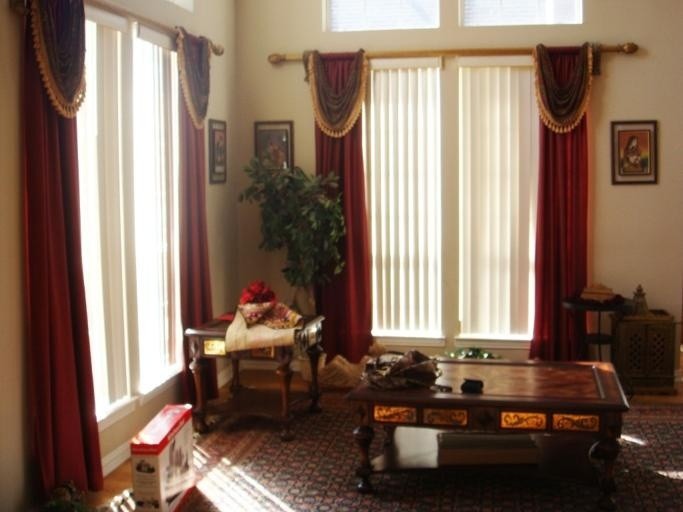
<box><xmin>254</xmin><ymin>120</ymin><xmax>294</xmax><ymax>184</ymax></box>
<box><xmin>611</xmin><ymin>120</ymin><xmax>658</xmax><ymax>184</ymax></box>
<box><xmin>208</xmin><ymin>118</ymin><xmax>226</xmax><ymax>185</ymax></box>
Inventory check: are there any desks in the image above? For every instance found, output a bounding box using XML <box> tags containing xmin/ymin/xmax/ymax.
<box><xmin>184</xmin><ymin>313</ymin><xmax>327</xmax><ymax>440</ymax></box>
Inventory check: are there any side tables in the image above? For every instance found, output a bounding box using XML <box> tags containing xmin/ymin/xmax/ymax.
<box><xmin>562</xmin><ymin>297</ymin><xmax>635</xmax><ymax>401</ymax></box>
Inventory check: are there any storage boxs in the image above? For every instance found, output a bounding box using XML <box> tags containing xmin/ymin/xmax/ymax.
<box><xmin>129</xmin><ymin>402</ymin><xmax>194</xmax><ymax>512</ymax></box>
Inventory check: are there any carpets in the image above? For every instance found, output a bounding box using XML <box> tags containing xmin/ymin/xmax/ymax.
<box><xmin>167</xmin><ymin>389</ymin><xmax>683</xmax><ymax>512</ymax></box>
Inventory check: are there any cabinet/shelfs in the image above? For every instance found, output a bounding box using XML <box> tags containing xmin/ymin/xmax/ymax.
<box><xmin>608</xmin><ymin>309</ymin><xmax>677</xmax><ymax>395</ymax></box>
